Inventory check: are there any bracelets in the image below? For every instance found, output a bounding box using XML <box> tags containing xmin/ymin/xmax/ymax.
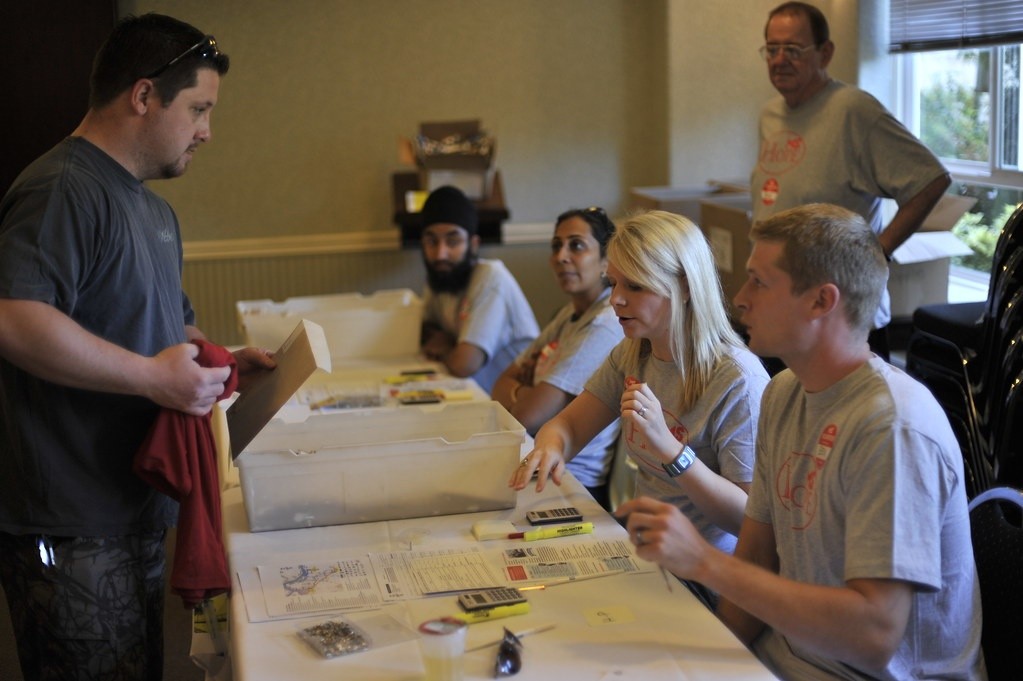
<box><xmin>510</xmin><ymin>381</ymin><xmax>524</xmax><ymax>405</ymax></box>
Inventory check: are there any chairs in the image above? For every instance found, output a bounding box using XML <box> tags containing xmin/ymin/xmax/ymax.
<box><xmin>898</xmin><ymin>200</ymin><xmax>1023</xmax><ymax>681</ymax></box>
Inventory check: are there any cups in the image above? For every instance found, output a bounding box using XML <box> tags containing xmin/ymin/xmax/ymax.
<box><xmin>415</xmin><ymin>616</ymin><xmax>468</xmax><ymax>681</ymax></box>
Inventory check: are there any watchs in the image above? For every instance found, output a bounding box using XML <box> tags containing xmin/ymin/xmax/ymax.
<box><xmin>661</xmin><ymin>443</ymin><xmax>696</xmax><ymax>478</ymax></box>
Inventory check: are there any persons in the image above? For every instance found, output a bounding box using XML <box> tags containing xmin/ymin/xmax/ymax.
<box><xmin>2</xmin><ymin>14</ymin><xmax>280</xmax><ymax>681</ymax></box>
<box><xmin>504</xmin><ymin>209</ymin><xmax>776</xmax><ymax>612</ymax></box>
<box><xmin>488</xmin><ymin>210</ymin><xmax>632</xmax><ymax>513</ymax></box>
<box><xmin>613</xmin><ymin>203</ymin><xmax>989</xmax><ymax>680</ymax></box>
<box><xmin>745</xmin><ymin>2</ymin><xmax>954</xmax><ymax>377</ymax></box>
<box><xmin>417</xmin><ymin>185</ymin><xmax>544</xmax><ymax>397</ymax></box>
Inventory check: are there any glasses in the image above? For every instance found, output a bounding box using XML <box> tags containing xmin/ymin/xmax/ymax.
<box><xmin>583</xmin><ymin>205</ymin><xmax>614</xmax><ymax>240</ymax></box>
<box><xmin>755</xmin><ymin>41</ymin><xmax>819</xmax><ymax>62</ymax></box>
<box><xmin>140</xmin><ymin>34</ymin><xmax>219</xmax><ymax>78</ymax></box>
<box><xmin>491</xmin><ymin>626</ymin><xmax>526</xmax><ymax>679</ymax></box>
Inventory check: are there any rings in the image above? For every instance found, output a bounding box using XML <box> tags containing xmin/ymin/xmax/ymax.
<box><xmin>637</xmin><ymin>405</ymin><xmax>648</xmax><ymax>417</ymax></box>
<box><xmin>520</xmin><ymin>459</ymin><xmax>528</xmax><ymax>466</ymax></box>
<box><xmin>636</xmin><ymin>529</ymin><xmax>644</xmax><ymax>545</ymax></box>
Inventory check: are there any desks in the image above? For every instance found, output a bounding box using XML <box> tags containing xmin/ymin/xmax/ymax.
<box><xmin>218</xmin><ymin>356</ymin><xmax>558</xmax><ymax>485</ymax></box>
<box><xmin>213</xmin><ymin>474</ymin><xmax>790</xmax><ymax>681</ymax></box>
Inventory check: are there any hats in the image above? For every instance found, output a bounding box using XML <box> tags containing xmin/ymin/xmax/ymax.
<box><xmin>420</xmin><ymin>184</ymin><xmax>475</xmax><ymax>234</ymax></box>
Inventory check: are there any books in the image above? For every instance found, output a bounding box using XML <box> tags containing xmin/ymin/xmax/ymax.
<box><xmin>226</xmin><ymin>318</ymin><xmax>331</xmax><ymax>467</ymax></box>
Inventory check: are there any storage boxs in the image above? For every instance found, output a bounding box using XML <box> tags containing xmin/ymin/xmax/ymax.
<box><xmin>386</xmin><ymin>172</ymin><xmax>513</xmax><ymax>249</ymax></box>
<box><xmin>886</xmin><ymin>184</ymin><xmax>982</xmax><ymax>317</ymax></box>
<box><xmin>693</xmin><ymin>192</ymin><xmax>752</xmax><ymax>297</ymax></box>
<box><xmin>406</xmin><ymin>117</ymin><xmax>499</xmax><ymax>200</ymax></box>
<box><xmin>234</xmin><ymin>286</ymin><xmax>426</xmax><ymax>363</ymax></box>
<box><xmin>231</xmin><ymin>398</ymin><xmax>528</xmax><ymax>535</ymax></box>
<box><xmin>624</xmin><ymin>181</ymin><xmax>722</xmax><ymax>224</ymax></box>
